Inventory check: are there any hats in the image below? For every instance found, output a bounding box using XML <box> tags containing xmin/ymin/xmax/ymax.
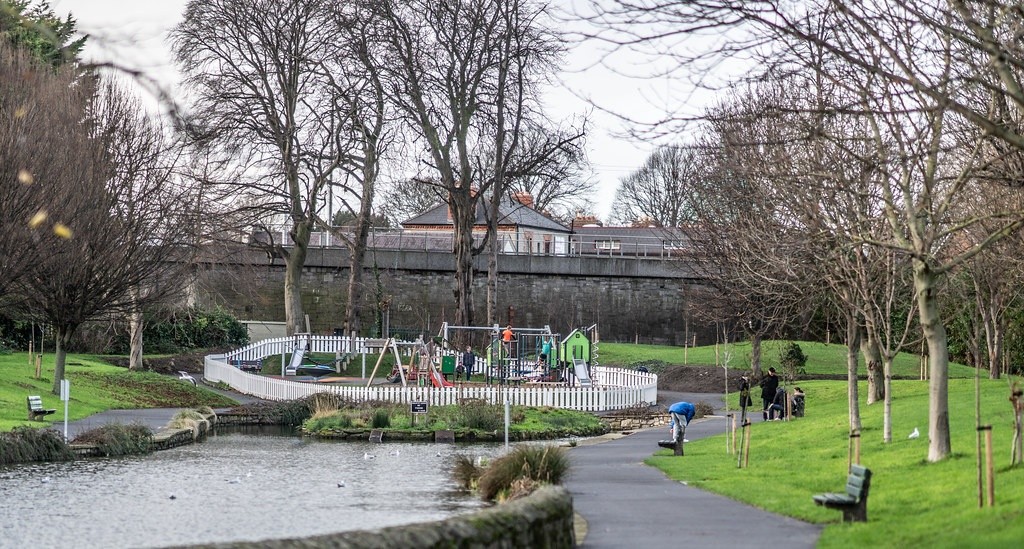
<box><xmin>769</xmin><ymin>367</ymin><xmax>776</xmax><ymax>373</ymax></box>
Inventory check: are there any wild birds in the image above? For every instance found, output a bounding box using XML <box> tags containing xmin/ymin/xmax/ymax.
<box><xmin>909</xmin><ymin>426</ymin><xmax>919</xmax><ymax>440</ymax></box>
<box><xmin>177</xmin><ymin>370</ymin><xmax>197</xmax><ymax>387</ymax></box>
<box><xmin>390</xmin><ymin>449</ymin><xmax>401</xmax><ymax>456</ymax></box>
<box><xmin>170</xmin><ymin>495</ymin><xmax>176</xmax><ymax>499</ymax></box>
<box><xmin>338</xmin><ymin>481</ymin><xmax>345</xmax><ymax>487</ymax></box>
<box><xmin>364</xmin><ymin>451</ymin><xmax>376</xmax><ymax>461</ymax></box>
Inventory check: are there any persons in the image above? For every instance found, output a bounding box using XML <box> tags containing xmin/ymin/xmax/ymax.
<box><xmin>500</xmin><ymin>325</ymin><xmax>516</xmax><ymax>359</ymax></box>
<box><xmin>637</xmin><ymin>364</ymin><xmax>648</xmax><ymax>375</ymax></box>
<box><xmin>456</xmin><ymin>362</ymin><xmax>464</xmax><ymax>381</ymax></box>
<box><xmin>737</xmin><ymin>371</ymin><xmax>752</xmax><ymax>426</ymax></box>
<box><xmin>669</xmin><ymin>401</ymin><xmax>695</xmax><ymax>443</ymax></box>
<box><xmin>462</xmin><ymin>345</ymin><xmax>475</xmax><ymax>382</ymax></box>
<box><xmin>791</xmin><ymin>387</ymin><xmax>805</xmax><ymax>417</ymax></box>
<box><xmin>759</xmin><ymin>367</ymin><xmax>787</xmax><ymax>420</ymax></box>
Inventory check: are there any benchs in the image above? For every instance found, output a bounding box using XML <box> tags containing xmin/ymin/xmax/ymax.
<box><xmin>814</xmin><ymin>464</ymin><xmax>872</xmax><ymax>525</ymax></box>
<box><xmin>27</xmin><ymin>395</ymin><xmax>57</xmax><ymax>422</ymax></box>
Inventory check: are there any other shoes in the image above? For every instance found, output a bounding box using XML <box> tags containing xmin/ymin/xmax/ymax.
<box><xmin>766</xmin><ymin>418</ymin><xmax>781</xmax><ymax>421</ymax></box>
<box><xmin>683</xmin><ymin>439</ymin><xmax>689</xmax><ymax>442</ymax></box>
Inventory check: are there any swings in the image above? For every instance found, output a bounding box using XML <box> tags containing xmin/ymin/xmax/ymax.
<box><xmin>386</xmin><ymin>346</ymin><xmax>407</xmax><ymax>384</ymax></box>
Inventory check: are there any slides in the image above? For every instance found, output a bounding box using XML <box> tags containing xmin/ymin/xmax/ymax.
<box><xmin>430</xmin><ymin>371</ymin><xmax>453</xmax><ymax>387</ymax></box>
<box><xmin>572</xmin><ymin>358</ymin><xmax>592</xmax><ymax>389</ymax></box>
